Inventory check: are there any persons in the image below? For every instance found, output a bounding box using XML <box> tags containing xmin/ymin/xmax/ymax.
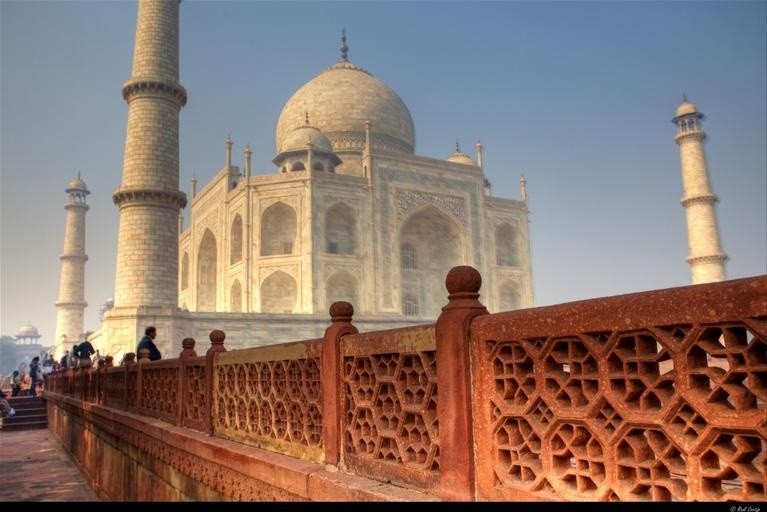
<box><xmin>0</xmin><ymin>337</ymin><xmax>94</xmax><ymax>431</ymax></box>
<box><xmin>137</xmin><ymin>326</ymin><xmax>161</xmax><ymax>361</ymax></box>
